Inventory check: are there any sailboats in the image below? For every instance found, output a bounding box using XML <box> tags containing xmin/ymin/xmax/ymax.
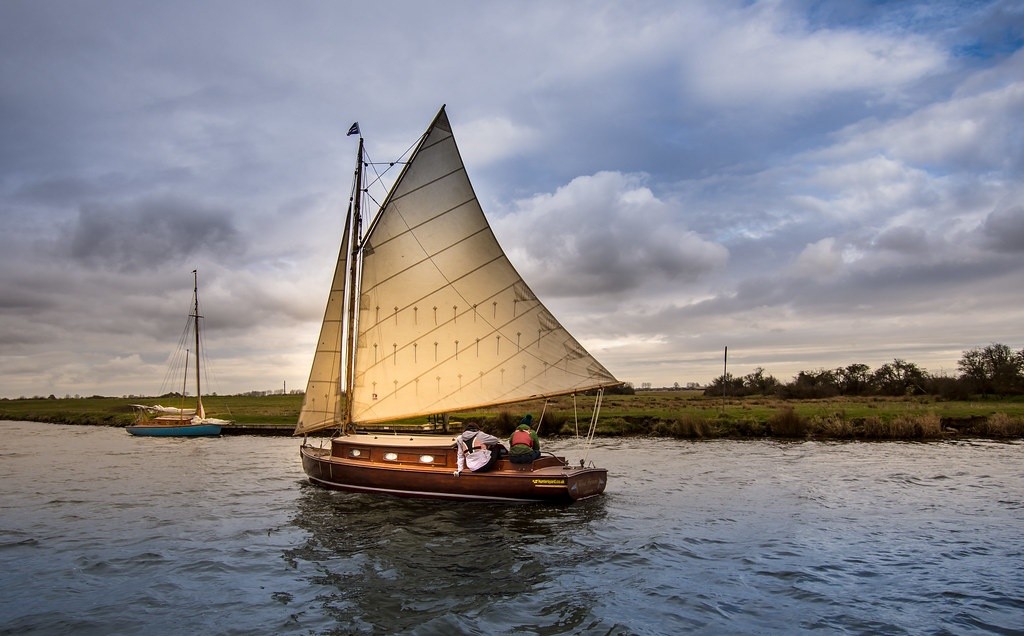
<box><xmin>290</xmin><ymin>103</ymin><xmax>628</xmax><ymax>507</ymax></box>
<box><xmin>124</xmin><ymin>269</ymin><xmax>237</xmax><ymax>437</ymax></box>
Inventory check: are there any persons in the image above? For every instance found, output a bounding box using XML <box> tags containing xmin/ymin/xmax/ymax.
<box><xmin>509</xmin><ymin>414</ymin><xmax>541</xmax><ymax>464</ymax></box>
<box><xmin>453</xmin><ymin>422</ymin><xmax>503</xmax><ymax>478</ymax></box>
